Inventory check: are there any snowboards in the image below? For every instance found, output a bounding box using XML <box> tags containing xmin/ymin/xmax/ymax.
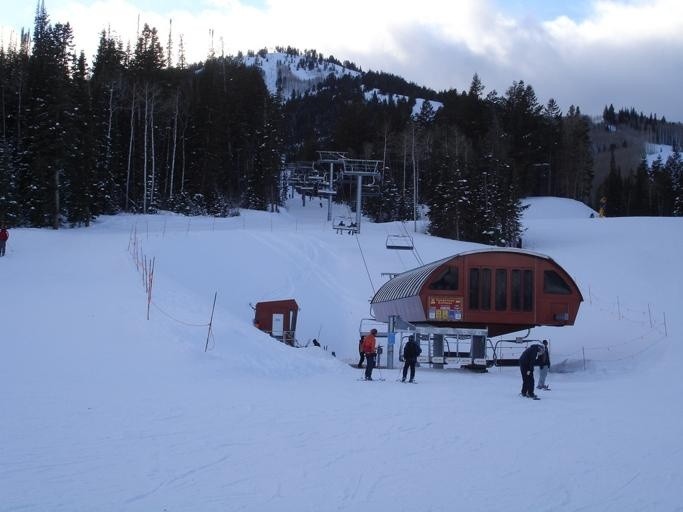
<box><xmin>520</xmin><ymin>390</ymin><xmax>542</xmax><ymax>401</ymax></box>
<box><xmin>537</xmin><ymin>384</ymin><xmax>551</xmax><ymax>391</ymax></box>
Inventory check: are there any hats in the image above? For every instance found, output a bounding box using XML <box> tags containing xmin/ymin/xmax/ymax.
<box><xmin>538</xmin><ymin>340</ymin><xmax>548</xmax><ymax>353</ymax></box>
<box><xmin>409</xmin><ymin>335</ymin><xmax>414</xmax><ymax>341</ymax></box>
<box><xmin>371</xmin><ymin>329</ymin><xmax>377</xmax><ymax>333</ymax></box>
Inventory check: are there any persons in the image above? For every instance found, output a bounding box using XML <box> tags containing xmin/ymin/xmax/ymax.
<box><xmin>312</xmin><ymin>338</ymin><xmax>320</xmax><ymax>347</ymax></box>
<box><xmin>537</xmin><ymin>339</ymin><xmax>551</xmax><ymax>388</ymax></box>
<box><xmin>357</xmin><ymin>335</ymin><xmax>365</xmax><ymax>369</ymax></box>
<box><xmin>287</xmin><ymin>193</ymin><xmax>358</xmax><ymax>236</ymax></box>
<box><xmin>598</xmin><ymin>196</ymin><xmax>608</xmax><ymax>217</ymax></box>
<box><xmin>361</xmin><ymin>328</ymin><xmax>377</xmax><ymax>381</ymax></box>
<box><xmin>401</xmin><ymin>334</ymin><xmax>423</xmax><ymax>383</ymax></box>
<box><xmin>519</xmin><ymin>343</ymin><xmax>545</xmax><ymax>397</ymax></box>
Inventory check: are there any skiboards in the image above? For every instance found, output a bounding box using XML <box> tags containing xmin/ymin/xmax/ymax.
<box><xmin>396</xmin><ymin>378</ymin><xmax>417</xmax><ymax>384</ymax></box>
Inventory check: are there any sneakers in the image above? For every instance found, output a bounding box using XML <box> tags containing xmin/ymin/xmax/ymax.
<box><xmin>538</xmin><ymin>385</ymin><xmax>546</xmax><ymax>389</ymax></box>
<box><xmin>365</xmin><ymin>376</ymin><xmax>372</xmax><ymax>380</ymax></box>
<box><xmin>522</xmin><ymin>393</ymin><xmax>537</xmax><ymax>398</ymax></box>
<box><xmin>402</xmin><ymin>377</ymin><xmax>413</xmax><ymax>382</ymax></box>
